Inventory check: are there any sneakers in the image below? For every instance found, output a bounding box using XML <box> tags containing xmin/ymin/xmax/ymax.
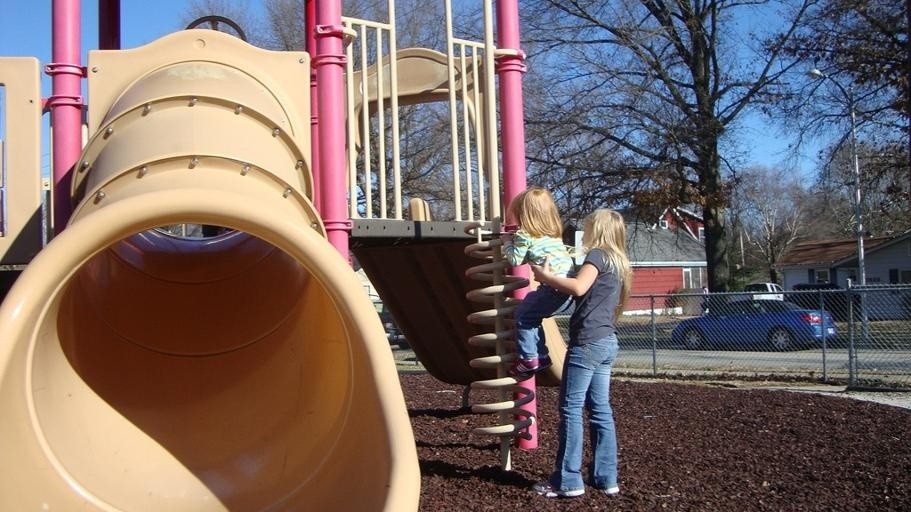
<box><xmin>534</xmin><ymin>355</ymin><xmax>552</xmax><ymax>373</ymax></box>
<box><xmin>509</xmin><ymin>358</ymin><xmax>539</xmax><ymax>374</ymax></box>
<box><xmin>534</xmin><ymin>480</ymin><xmax>585</xmax><ymax>497</ymax></box>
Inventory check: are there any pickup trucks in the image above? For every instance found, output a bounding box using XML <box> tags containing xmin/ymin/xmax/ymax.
<box><xmin>728</xmin><ymin>280</ymin><xmax>786</xmax><ymax>302</ymax></box>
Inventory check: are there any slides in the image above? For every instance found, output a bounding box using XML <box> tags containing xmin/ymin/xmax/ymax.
<box><xmin>349</xmin><ymin>196</ymin><xmax>568</xmax><ymax>388</ymax></box>
<box><xmin>1</xmin><ymin>27</ymin><xmax>421</xmax><ymax>512</ymax></box>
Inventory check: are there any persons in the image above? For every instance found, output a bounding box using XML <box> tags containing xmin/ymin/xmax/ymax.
<box><xmin>529</xmin><ymin>207</ymin><xmax>633</xmax><ymax>498</ymax></box>
<box><xmin>500</xmin><ymin>187</ymin><xmax>576</xmax><ymax>378</ymax></box>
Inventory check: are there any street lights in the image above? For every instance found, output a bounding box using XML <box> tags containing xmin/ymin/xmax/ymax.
<box><xmin>804</xmin><ymin>63</ymin><xmax>874</xmax><ymax>341</ymax></box>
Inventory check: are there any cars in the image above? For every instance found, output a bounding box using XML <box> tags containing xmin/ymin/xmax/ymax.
<box><xmin>670</xmin><ymin>297</ymin><xmax>836</xmax><ymax>352</ymax></box>
